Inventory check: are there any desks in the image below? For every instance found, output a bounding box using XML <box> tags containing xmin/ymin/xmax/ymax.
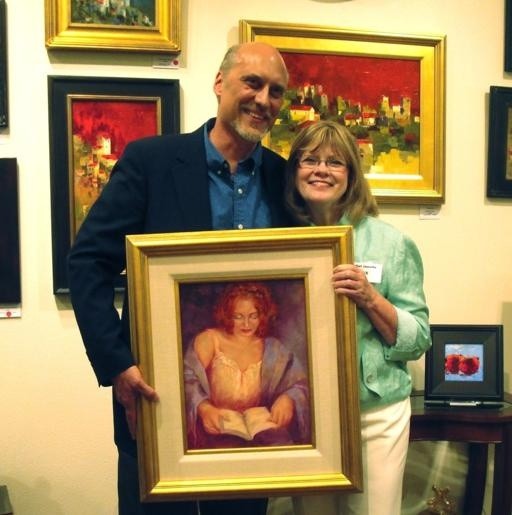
<box><xmin>401</xmin><ymin>390</ymin><xmax>511</xmax><ymax>515</ymax></box>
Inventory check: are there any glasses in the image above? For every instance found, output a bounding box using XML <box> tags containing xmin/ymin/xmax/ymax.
<box><xmin>295</xmin><ymin>155</ymin><xmax>349</xmax><ymax>173</ymax></box>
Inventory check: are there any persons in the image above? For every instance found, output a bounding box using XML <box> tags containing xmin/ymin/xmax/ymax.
<box><xmin>284</xmin><ymin>119</ymin><xmax>432</xmax><ymax>514</ymax></box>
<box><xmin>183</xmin><ymin>282</ymin><xmax>311</xmax><ymax>450</ymax></box>
<box><xmin>63</xmin><ymin>41</ymin><xmax>311</xmax><ymax>515</ymax></box>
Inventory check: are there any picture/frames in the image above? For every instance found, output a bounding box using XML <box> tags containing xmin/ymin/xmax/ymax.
<box><xmin>123</xmin><ymin>224</ymin><xmax>365</xmax><ymax>507</ymax></box>
<box><xmin>43</xmin><ymin>0</ymin><xmax>184</xmax><ymax>56</ymax></box>
<box><xmin>482</xmin><ymin>82</ymin><xmax>512</xmax><ymax>203</ymax></box>
<box><xmin>421</xmin><ymin>321</ymin><xmax>505</xmax><ymax>409</ymax></box>
<box><xmin>499</xmin><ymin>0</ymin><xmax>511</xmax><ymax>75</ymax></box>
<box><xmin>0</xmin><ymin>156</ymin><xmax>25</xmax><ymax>319</ymax></box>
<box><xmin>236</xmin><ymin>17</ymin><xmax>449</xmax><ymax>207</ymax></box>
<box><xmin>46</xmin><ymin>72</ymin><xmax>183</xmax><ymax>299</ymax></box>
<box><xmin>0</xmin><ymin>0</ymin><xmax>12</xmax><ymax>130</ymax></box>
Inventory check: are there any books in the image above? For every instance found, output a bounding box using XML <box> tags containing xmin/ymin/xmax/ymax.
<box><xmin>218</xmin><ymin>406</ymin><xmax>278</xmax><ymax>441</ymax></box>
